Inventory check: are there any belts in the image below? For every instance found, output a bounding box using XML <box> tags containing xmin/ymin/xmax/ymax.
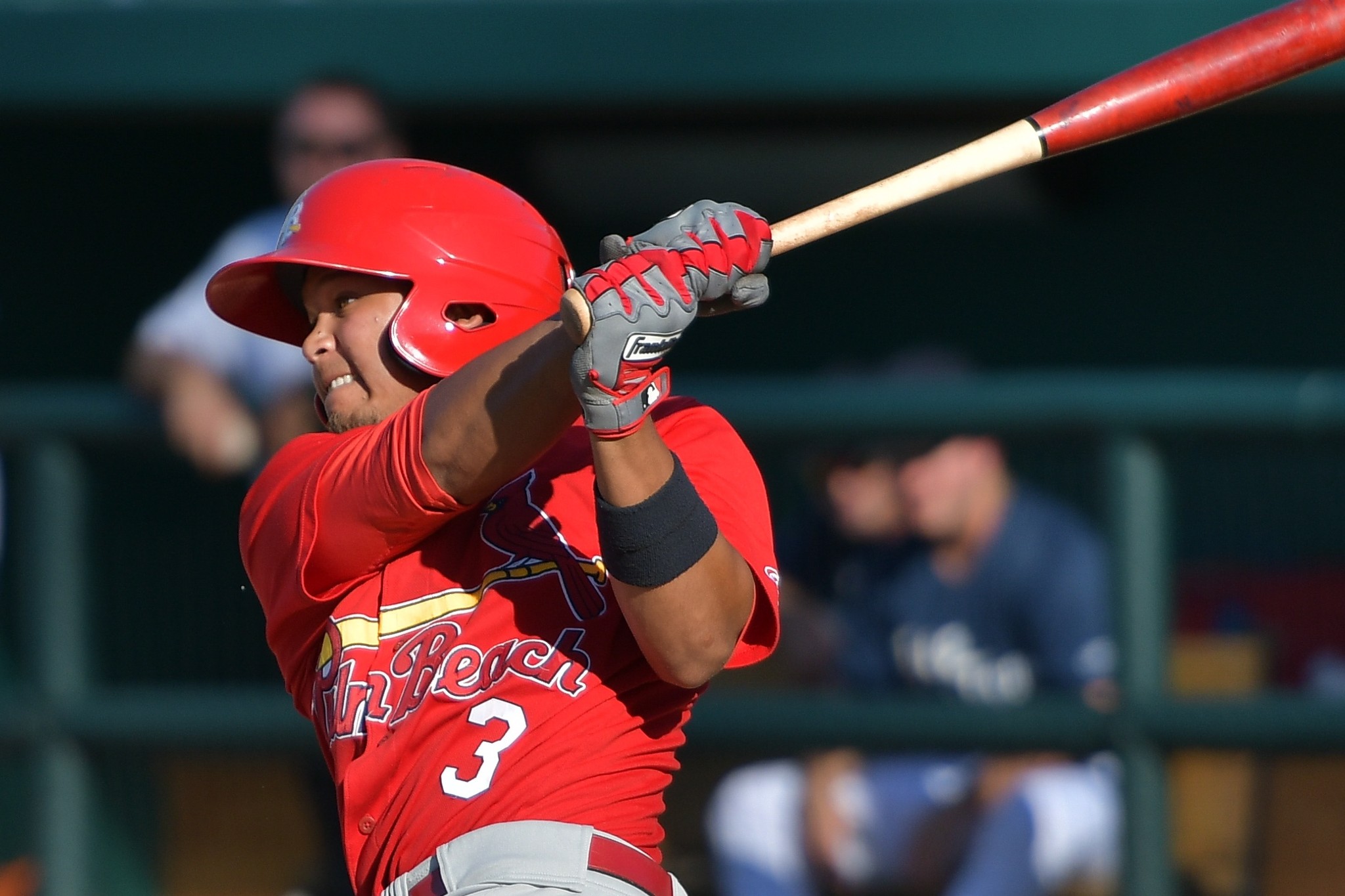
<box><xmin>408</xmin><ymin>834</ymin><xmax>674</xmax><ymax>896</ymax></box>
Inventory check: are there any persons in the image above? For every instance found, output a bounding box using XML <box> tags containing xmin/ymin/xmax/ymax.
<box><xmin>132</xmin><ymin>86</ymin><xmax>396</xmax><ymax>474</ymax></box>
<box><xmin>203</xmin><ymin>156</ymin><xmax>780</xmax><ymax>896</ymax></box>
<box><xmin>709</xmin><ymin>432</ymin><xmax>1123</xmax><ymax>896</ymax></box>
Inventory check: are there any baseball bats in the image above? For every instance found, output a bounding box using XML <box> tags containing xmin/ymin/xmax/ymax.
<box><xmin>558</xmin><ymin>0</ymin><xmax>1344</xmax><ymax>346</ymax></box>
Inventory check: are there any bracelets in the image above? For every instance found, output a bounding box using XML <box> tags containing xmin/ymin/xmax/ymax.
<box><xmin>592</xmin><ymin>449</ymin><xmax>719</xmax><ymax>588</ymax></box>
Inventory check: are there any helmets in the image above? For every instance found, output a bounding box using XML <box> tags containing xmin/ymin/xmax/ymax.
<box><xmin>206</xmin><ymin>160</ymin><xmax>578</xmax><ymax>380</ymax></box>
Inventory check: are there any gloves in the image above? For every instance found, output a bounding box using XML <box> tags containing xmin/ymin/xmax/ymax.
<box><xmin>569</xmin><ymin>239</ymin><xmax>701</xmax><ymax>440</ymax></box>
<box><xmin>598</xmin><ymin>198</ymin><xmax>771</xmax><ymax>318</ymax></box>
